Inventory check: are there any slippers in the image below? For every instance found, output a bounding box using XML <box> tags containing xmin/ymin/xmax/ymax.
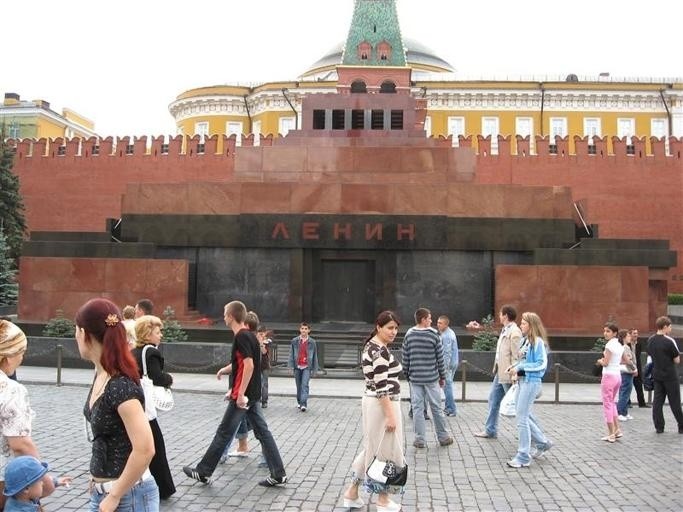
<box><xmin>376</xmin><ymin>499</ymin><xmax>402</xmax><ymax>512</ymax></box>
<box><xmin>344</xmin><ymin>496</ymin><xmax>363</xmax><ymax>508</ymax></box>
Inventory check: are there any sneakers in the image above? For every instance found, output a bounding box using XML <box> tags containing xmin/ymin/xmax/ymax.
<box><xmin>601</xmin><ymin>414</ymin><xmax>633</xmax><ymax>442</ymax></box>
<box><xmin>183</xmin><ymin>467</ymin><xmax>211</xmax><ymax>484</ymax></box>
<box><xmin>476</xmin><ymin>432</ymin><xmax>497</xmax><ymax>437</ymax></box>
<box><xmin>532</xmin><ymin>440</ymin><xmax>552</xmax><ymax>459</ymax></box>
<box><xmin>259</xmin><ymin>475</ymin><xmax>288</xmax><ymax>486</ymax></box>
<box><xmin>507</xmin><ymin>458</ymin><xmax>531</xmax><ymax>467</ymax></box>
<box><xmin>410</xmin><ymin>407</ymin><xmax>456</xmax><ymax>447</ymax></box>
<box><xmin>228</xmin><ymin>450</ymin><xmax>249</xmax><ymax>457</ymax></box>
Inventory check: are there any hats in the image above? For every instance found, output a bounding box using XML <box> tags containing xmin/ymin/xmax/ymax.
<box><xmin>3</xmin><ymin>456</ymin><xmax>47</xmax><ymax>497</ymax></box>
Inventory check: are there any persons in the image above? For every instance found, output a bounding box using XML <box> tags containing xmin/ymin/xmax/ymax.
<box><xmin>403</xmin><ymin>308</ymin><xmax>458</xmax><ymax>448</ymax></box>
<box><xmin>216</xmin><ymin>311</ymin><xmax>273</xmax><ymax>467</ymax></box>
<box><xmin>599</xmin><ymin>316</ymin><xmax>682</xmax><ymax>442</ymax></box>
<box><xmin>0</xmin><ymin>320</ymin><xmax>55</xmax><ymax>512</ymax></box>
<box><xmin>342</xmin><ymin>311</ymin><xmax>404</xmax><ymax>512</ymax></box>
<box><xmin>476</xmin><ymin>305</ymin><xmax>523</xmax><ymax>440</ymax></box>
<box><xmin>506</xmin><ymin>311</ymin><xmax>552</xmax><ymax>468</ymax></box>
<box><xmin>4</xmin><ymin>456</ymin><xmax>73</xmax><ymax>512</ymax></box>
<box><xmin>121</xmin><ymin>299</ymin><xmax>177</xmax><ymax>500</ymax></box>
<box><xmin>75</xmin><ymin>298</ymin><xmax>161</xmax><ymax>512</ymax></box>
<box><xmin>288</xmin><ymin>322</ymin><xmax>319</xmax><ymax>412</ymax></box>
<box><xmin>183</xmin><ymin>300</ymin><xmax>288</xmax><ymax>487</ymax></box>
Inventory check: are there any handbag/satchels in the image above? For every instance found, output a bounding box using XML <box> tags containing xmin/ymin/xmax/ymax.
<box><xmin>140</xmin><ymin>379</ymin><xmax>156</xmax><ymax>421</ymax></box>
<box><xmin>644</xmin><ymin>363</ymin><xmax>654</xmax><ymax>391</ymax></box>
<box><xmin>365</xmin><ymin>458</ymin><xmax>408</xmax><ymax>493</ymax></box>
<box><xmin>142</xmin><ymin>374</ymin><xmax>174</xmax><ymax>411</ymax></box>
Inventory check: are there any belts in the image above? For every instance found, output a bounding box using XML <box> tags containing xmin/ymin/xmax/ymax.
<box><xmin>90</xmin><ymin>467</ymin><xmax>151</xmax><ymax>495</ymax></box>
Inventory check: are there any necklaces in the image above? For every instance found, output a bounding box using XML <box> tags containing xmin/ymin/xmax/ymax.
<box><xmin>93</xmin><ymin>372</ymin><xmax>108</xmax><ymax>398</ymax></box>
